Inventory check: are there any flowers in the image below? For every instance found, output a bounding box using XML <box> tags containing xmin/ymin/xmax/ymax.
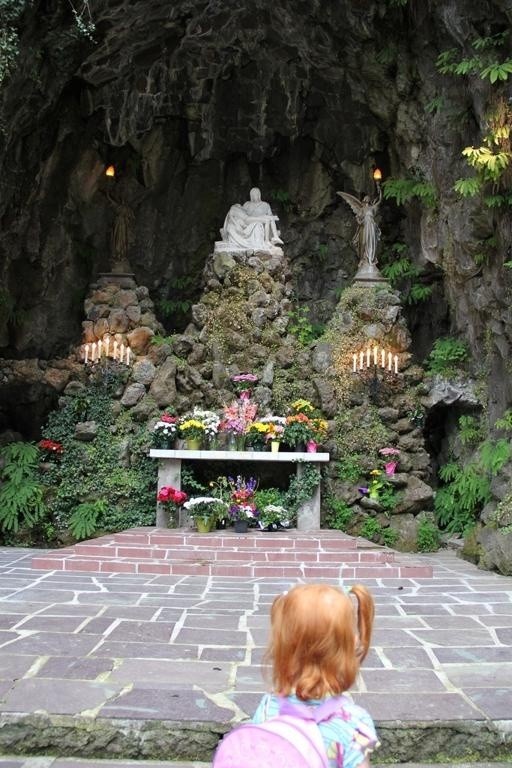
<box><xmin>154</xmin><ymin>398</ymin><xmax>329</xmax><ymax>439</ymax></box>
<box><xmin>158</xmin><ymin>476</ymin><xmax>289</xmax><ymax>515</ymax></box>
<box><xmin>231</xmin><ymin>371</ymin><xmax>258</xmax><ymax>387</ymax></box>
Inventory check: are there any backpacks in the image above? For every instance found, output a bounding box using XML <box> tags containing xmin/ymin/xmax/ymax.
<box><xmin>213</xmin><ymin>693</ymin><xmax>354</xmax><ymax>768</ymax></box>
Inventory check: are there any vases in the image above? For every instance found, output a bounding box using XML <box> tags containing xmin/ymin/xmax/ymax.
<box><xmin>166</xmin><ymin>513</ymin><xmax>278</xmax><ymax>533</ymax></box>
<box><xmin>240</xmin><ymin>385</ymin><xmax>250</xmax><ymax>400</ymax></box>
<box><xmin>158</xmin><ymin>434</ymin><xmax>315</xmax><ymax>453</ymax></box>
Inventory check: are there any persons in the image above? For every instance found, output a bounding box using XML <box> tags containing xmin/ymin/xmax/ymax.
<box><xmin>212</xmin><ymin>583</ymin><xmax>381</xmax><ymax>768</ymax></box>
